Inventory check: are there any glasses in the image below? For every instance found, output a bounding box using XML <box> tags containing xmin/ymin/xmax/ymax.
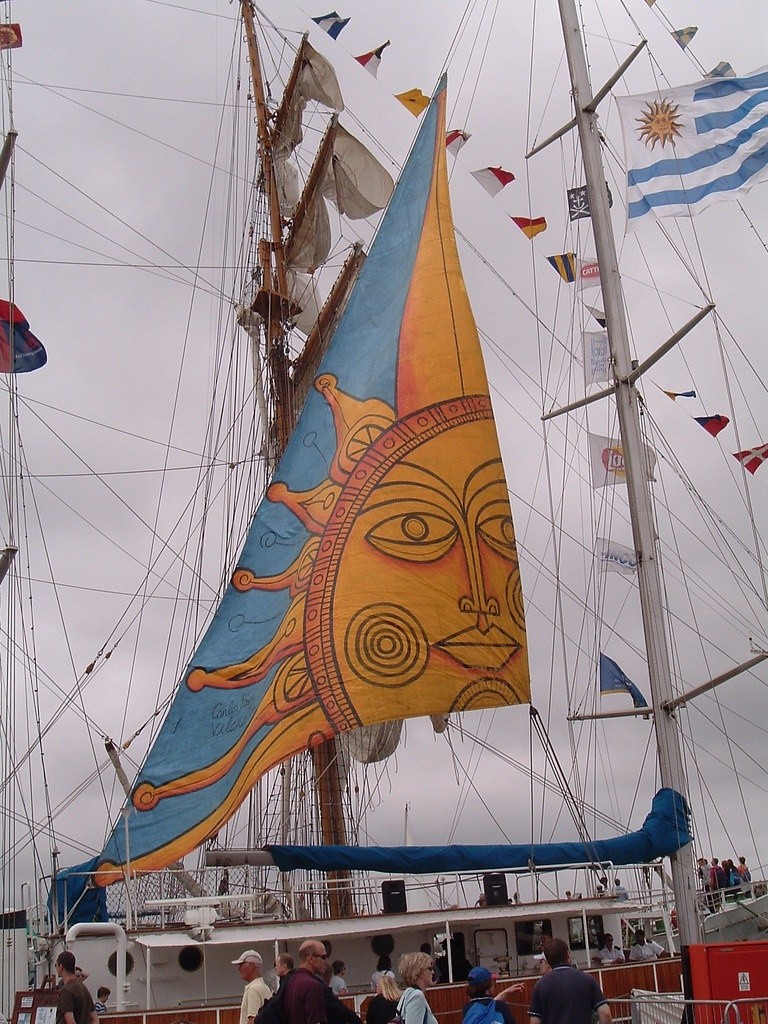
<box><xmin>607</xmin><ymin>938</ymin><xmax>614</xmax><ymax>942</ymax></box>
<box><xmin>306</xmin><ymin>952</ymin><xmax>328</xmax><ymax>960</ymax></box>
<box><xmin>425</xmin><ymin>965</ymin><xmax>433</xmax><ymax>972</ymax></box>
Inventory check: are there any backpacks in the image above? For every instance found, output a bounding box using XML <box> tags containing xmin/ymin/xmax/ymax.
<box><xmin>714</xmin><ymin>868</ymin><xmax>725</xmax><ymax>888</ymax></box>
<box><xmin>740</xmin><ymin>865</ymin><xmax>751</xmax><ymax>882</ymax></box>
<box><xmin>254</xmin><ymin>973</ymin><xmax>292</xmax><ymax>1024</ymax></box>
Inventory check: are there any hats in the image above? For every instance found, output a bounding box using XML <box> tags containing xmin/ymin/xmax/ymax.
<box><xmin>467</xmin><ymin>967</ymin><xmax>495</xmax><ymax>986</ymax></box>
<box><xmin>533</xmin><ymin>950</ymin><xmax>548</xmax><ymax>963</ymax></box>
<box><xmin>232</xmin><ymin>949</ymin><xmax>262</xmax><ymax>965</ymax></box>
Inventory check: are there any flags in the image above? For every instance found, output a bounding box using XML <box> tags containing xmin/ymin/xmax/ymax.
<box><xmin>312</xmin><ymin>0</ymin><xmax>768</xmax><ymax>719</ymax></box>
<box><xmin>0</xmin><ymin>23</ymin><xmax>22</xmax><ymax>51</ymax></box>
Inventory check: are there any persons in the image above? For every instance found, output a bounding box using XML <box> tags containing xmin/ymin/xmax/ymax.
<box><xmin>231</xmin><ymin>857</ymin><xmax>751</xmax><ymax>1024</ymax></box>
<box><xmin>55</xmin><ymin>951</ymin><xmax>110</xmax><ymax>1024</ymax></box>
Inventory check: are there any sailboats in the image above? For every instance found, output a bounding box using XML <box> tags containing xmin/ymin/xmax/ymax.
<box><xmin>0</xmin><ymin>0</ymin><xmax>768</xmax><ymax>1024</ymax></box>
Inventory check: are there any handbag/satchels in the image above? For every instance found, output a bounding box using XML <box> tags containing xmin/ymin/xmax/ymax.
<box><xmin>462</xmin><ymin>1000</ymin><xmax>506</xmax><ymax>1024</ymax></box>
